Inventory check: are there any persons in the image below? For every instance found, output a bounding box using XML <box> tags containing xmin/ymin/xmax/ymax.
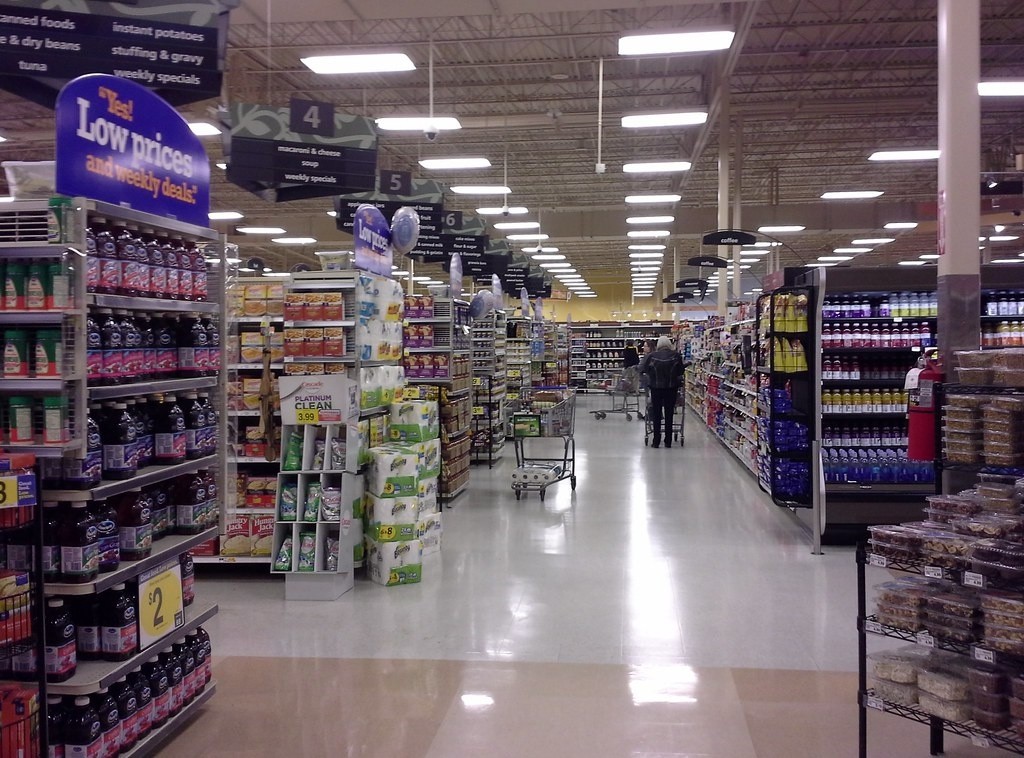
<box><xmin>633</xmin><ymin>340</ymin><xmax>657</xmax><ymax>420</ymax></box>
<box><xmin>643</xmin><ymin>335</ymin><xmax>685</xmax><ymax>449</ymax></box>
<box><xmin>623</xmin><ymin>340</ymin><xmax>640</xmax><ymax>369</ymax></box>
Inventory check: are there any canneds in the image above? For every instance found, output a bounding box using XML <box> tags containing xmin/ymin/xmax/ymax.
<box><xmin>0</xmin><ymin>197</ymin><xmax>75</xmax><ymax>447</ymax></box>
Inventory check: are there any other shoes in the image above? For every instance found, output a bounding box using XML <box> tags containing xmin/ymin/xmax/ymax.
<box><xmin>664</xmin><ymin>439</ymin><xmax>672</xmax><ymax>448</ymax></box>
<box><xmin>651</xmin><ymin>442</ymin><xmax>659</xmax><ymax>448</ymax></box>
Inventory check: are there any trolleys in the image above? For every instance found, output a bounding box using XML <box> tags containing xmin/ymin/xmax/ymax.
<box><xmin>644</xmin><ymin>374</ymin><xmax>685</xmax><ymax>446</ymax></box>
<box><xmin>511</xmin><ymin>385</ymin><xmax>579</xmax><ymax>501</ymax></box>
<box><xmin>589</xmin><ymin>365</ymin><xmax>644</xmax><ymax>421</ymax></box>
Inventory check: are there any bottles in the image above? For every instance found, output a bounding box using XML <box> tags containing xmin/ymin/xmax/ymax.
<box><xmin>0</xmin><ymin>208</ymin><xmax>212</xmax><ymax>758</ymax></box>
<box><xmin>819</xmin><ymin>288</ymin><xmax>1024</xmax><ymax>486</ymax></box>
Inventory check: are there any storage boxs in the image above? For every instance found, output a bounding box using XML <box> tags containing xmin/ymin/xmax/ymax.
<box><xmin>1</xmin><ymin>160</ymin><xmax>57</xmax><ymax>198</ymax></box>
<box><xmin>503</xmin><ymin>308</ymin><xmax>516</xmax><ymax>316</ymax></box>
<box><xmin>404</xmin><ymin>284</ymin><xmax>448</xmax><ymax>377</ymax></box>
<box><xmin>0</xmin><ymin>452</ymin><xmax>40</xmax><ymax>758</ymax></box>
<box><xmin>314</xmin><ymin>250</ymin><xmax>355</xmax><ymax>271</ymax></box>
<box><xmin>190</xmin><ymin>282</ymin><xmax>347</xmax><ymax>558</ymax></box>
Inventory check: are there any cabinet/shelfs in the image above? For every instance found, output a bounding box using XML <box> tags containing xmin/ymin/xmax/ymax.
<box><xmin>0</xmin><ymin>458</ymin><xmax>48</xmax><ymax>758</ymax></box>
<box><xmin>190</xmin><ymin>269</ymin><xmax>404</xmax><ymax>601</ymax></box>
<box><xmin>854</xmin><ymin>385</ymin><xmax>1024</xmax><ymax>758</ymax></box>
<box><xmin>471</xmin><ymin>266</ymin><xmax>1024</xmax><ymax>555</ymax></box>
<box><xmin>404</xmin><ymin>297</ymin><xmax>472</xmax><ymax>499</ymax></box>
<box><xmin>0</xmin><ymin>193</ymin><xmax>223</xmax><ymax>758</ymax></box>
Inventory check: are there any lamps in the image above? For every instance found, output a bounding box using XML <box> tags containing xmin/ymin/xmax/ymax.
<box><xmin>416</xmin><ymin>155</ymin><xmax>491</xmax><ymax>170</ymax></box>
<box><xmin>977</xmin><ymin>76</ymin><xmax>1024</xmax><ymax>97</ymax></box>
<box><xmin>616</xmin><ymin>23</ymin><xmax>735</xmax><ymax>55</ymax></box>
<box><xmin>622</xmin><ymin>158</ymin><xmax>692</xmax><ymax>173</ymax></box>
<box><xmin>987</xmin><ymin>179</ymin><xmax>998</xmax><ymax>188</ymax></box>
<box><xmin>868</xmin><ymin>147</ymin><xmax>940</xmax><ymax>160</ymax></box>
<box><xmin>186</xmin><ymin>118</ymin><xmax>221</xmax><ymax>136</ymax></box>
<box><xmin>375</xmin><ymin>113</ymin><xmax>462</xmax><ymax>131</ymax></box>
<box><xmin>299</xmin><ymin>46</ymin><xmax>419</xmax><ymax>74</ymax></box>
<box><xmin>992</xmin><ymin>198</ymin><xmax>1001</xmax><ymax>208</ymax></box>
<box><xmin>620</xmin><ymin>107</ymin><xmax>708</xmax><ymax>128</ymax></box>
